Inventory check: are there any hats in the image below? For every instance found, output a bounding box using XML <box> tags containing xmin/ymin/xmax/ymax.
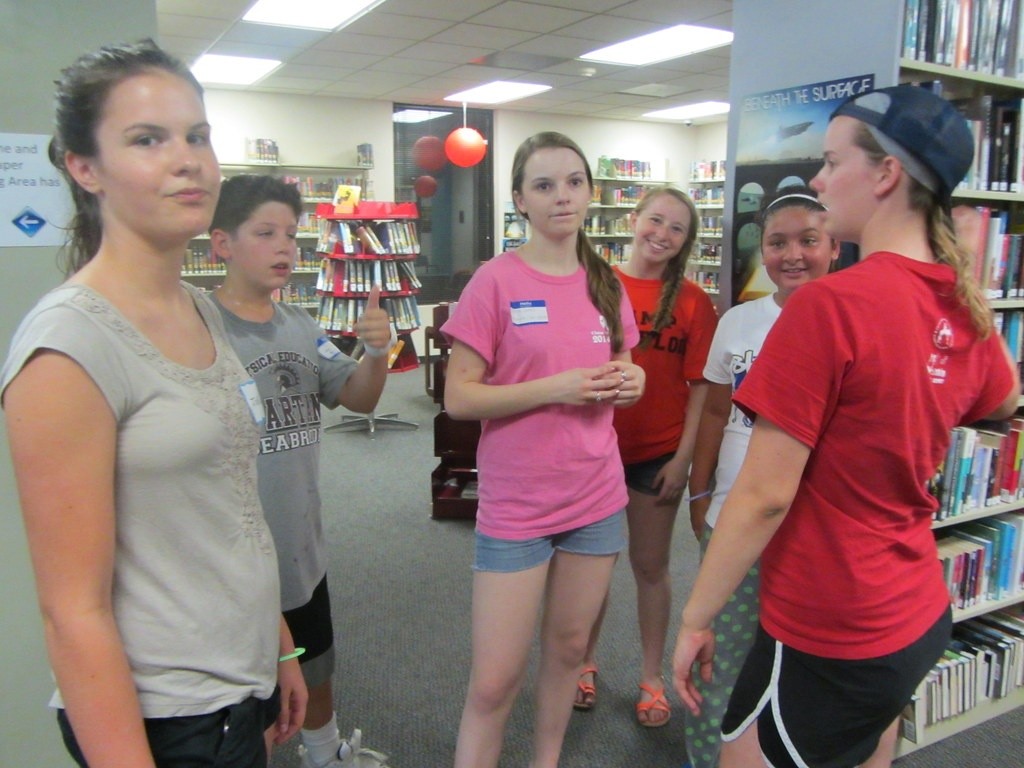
<box><xmin>829</xmin><ymin>85</ymin><xmax>976</xmax><ymax>217</ymax></box>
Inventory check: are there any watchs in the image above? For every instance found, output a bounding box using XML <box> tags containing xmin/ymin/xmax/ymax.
<box><xmin>364</xmin><ymin>328</ymin><xmax>398</xmax><ymax>358</ymax></box>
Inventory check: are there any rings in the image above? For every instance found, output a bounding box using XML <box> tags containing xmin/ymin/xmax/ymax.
<box><xmin>621</xmin><ymin>371</ymin><xmax>626</xmax><ymax>380</ymax></box>
<box><xmin>595</xmin><ymin>391</ymin><xmax>601</xmax><ymax>402</ymax></box>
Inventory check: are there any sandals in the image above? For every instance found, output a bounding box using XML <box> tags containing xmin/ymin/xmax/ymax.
<box><xmin>573</xmin><ymin>664</ymin><xmax>599</xmax><ymax>710</ymax></box>
<box><xmin>636</xmin><ymin>674</ymin><xmax>671</xmax><ymax>727</ymax></box>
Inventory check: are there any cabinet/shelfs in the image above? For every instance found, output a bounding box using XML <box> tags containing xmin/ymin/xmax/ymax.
<box><xmin>572</xmin><ymin>0</ymin><xmax>1024</xmax><ymax>762</ymax></box>
<box><xmin>179</xmin><ymin>163</ymin><xmax>481</xmax><ymax>522</ymax></box>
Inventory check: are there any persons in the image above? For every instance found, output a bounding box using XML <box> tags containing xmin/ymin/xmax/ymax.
<box><xmin>575</xmin><ymin>187</ymin><xmax>718</xmax><ymax>727</ymax></box>
<box><xmin>0</xmin><ymin>41</ymin><xmax>307</xmax><ymax>768</ymax></box>
<box><xmin>437</xmin><ymin>132</ymin><xmax>646</xmax><ymax>768</ymax></box>
<box><xmin>682</xmin><ymin>185</ymin><xmax>840</xmax><ymax>768</ymax></box>
<box><xmin>202</xmin><ymin>177</ymin><xmax>388</xmax><ymax>768</ymax></box>
<box><xmin>675</xmin><ymin>82</ymin><xmax>1021</xmax><ymax>768</ymax></box>
<box><xmin>438</xmin><ymin>134</ymin><xmax>649</xmax><ymax>768</ymax></box>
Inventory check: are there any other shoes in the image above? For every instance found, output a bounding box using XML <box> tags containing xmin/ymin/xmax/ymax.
<box><xmin>297</xmin><ymin>728</ymin><xmax>390</xmax><ymax>768</ymax></box>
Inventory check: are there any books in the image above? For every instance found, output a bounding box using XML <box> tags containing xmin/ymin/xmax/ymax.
<box><xmin>182</xmin><ymin>174</ymin><xmax>421</xmax><ymax>371</ymax></box>
<box><xmin>895</xmin><ymin>0</ymin><xmax>1024</xmax><ymax>746</ymax></box>
<box><xmin>584</xmin><ymin>154</ymin><xmax>727</xmax><ymax>292</ymax></box>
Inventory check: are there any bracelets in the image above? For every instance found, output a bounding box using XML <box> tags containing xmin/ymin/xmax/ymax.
<box><xmin>279</xmin><ymin>647</ymin><xmax>305</xmax><ymax>662</ymax></box>
<box><xmin>687</xmin><ymin>490</ymin><xmax>711</xmax><ymax>502</ymax></box>
